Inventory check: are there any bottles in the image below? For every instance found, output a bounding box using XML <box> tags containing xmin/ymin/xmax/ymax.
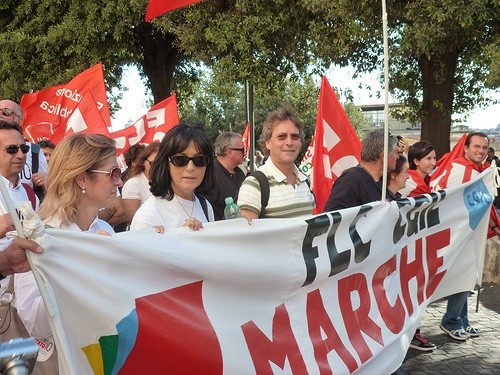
<box><xmin>224</xmin><ymin>197</ymin><xmax>243</xmax><ymax>220</ymax></box>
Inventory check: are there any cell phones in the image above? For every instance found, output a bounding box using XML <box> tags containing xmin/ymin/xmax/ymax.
<box><xmin>396</xmin><ymin>136</ymin><xmax>402</xmax><ymax>146</ymax></box>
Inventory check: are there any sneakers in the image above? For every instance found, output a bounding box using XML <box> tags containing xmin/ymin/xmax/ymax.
<box><xmin>409</xmin><ymin>330</ymin><xmax>436</xmax><ymax>351</ymax></box>
<box><xmin>440</xmin><ymin>320</ymin><xmax>480</xmax><ymax>340</ymax></box>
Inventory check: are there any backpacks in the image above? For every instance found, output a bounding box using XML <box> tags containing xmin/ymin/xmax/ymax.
<box><xmin>221</xmin><ymin>171</ymin><xmax>270</xmax><ymax>220</ymax></box>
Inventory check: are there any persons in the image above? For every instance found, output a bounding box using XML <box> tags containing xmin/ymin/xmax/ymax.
<box><xmin>0</xmin><ymin>100</ymin><xmax>500</xmax><ymax>375</ymax></box>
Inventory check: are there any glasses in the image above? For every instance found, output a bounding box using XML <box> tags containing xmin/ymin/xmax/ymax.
<box><xmin>168</xmin><ymin>154</ymin><xmax>210</xmax><ymax>167</ymax></box>
<box><xmin>146</xmin><ymin>158</ymin><xmax>152</xmax><ymax>165</ymax></box>
<box><xmin>89</xmin><ymin>168</ymin><xmax>121</xmax><ymax>183</ymax></box>
<box><xmin>3</xmin><ymin>144</ymin><xmax>29</xmax><ymax>154</ymax></box>
<box><xmin>0</xmin><ymin>108</ymin><xmax>20</xmax><ymax>117</ymax></box>
<box><xmin>229</xmin><ymin>147</ymin><xmax>244</xmax><ymax>153</ymax></box>
